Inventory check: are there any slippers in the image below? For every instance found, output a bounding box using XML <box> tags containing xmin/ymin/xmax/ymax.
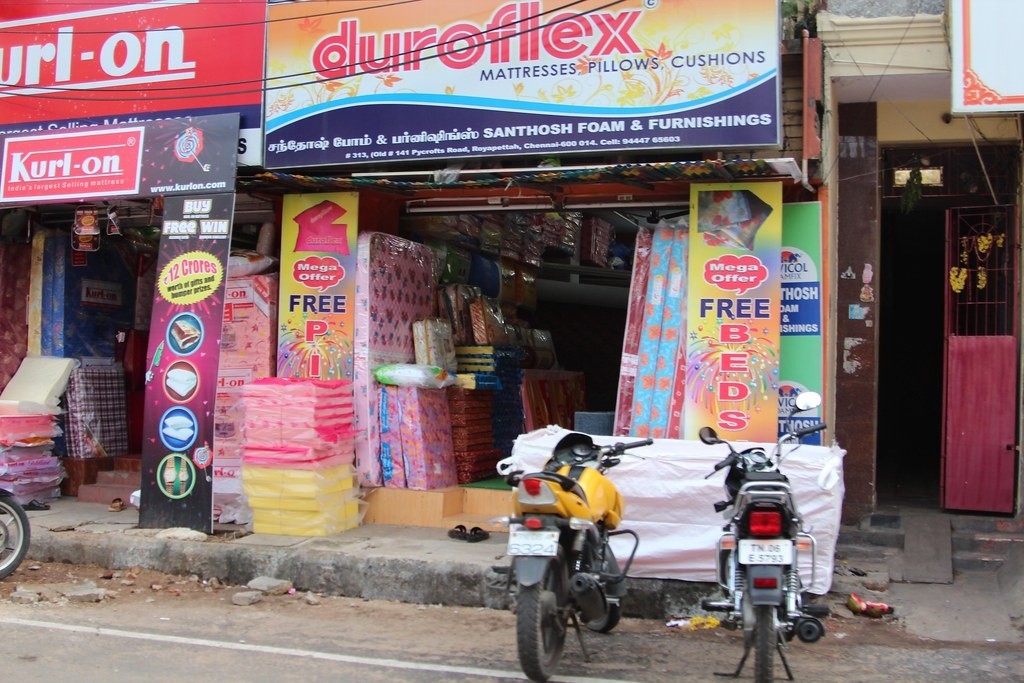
<box><xmin>448</xmin><ymin>525</ymin><xmax>469</xmax><ymax>540</ymax></box>
<box><xmin>108</xmin><ymin>497</ymin><xmax>127</xmax><ymax>512</ymax></box>
<box><xmin>466</xmin><ymin>527</ymin><xmax>489</xmax><ymax>543</ymax></box>
<box><xmin>21</xmin><ymin>499</ymin><xmax>50</xmax><ymax>510</ymax></box>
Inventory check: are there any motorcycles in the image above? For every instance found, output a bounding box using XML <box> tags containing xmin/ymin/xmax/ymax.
<box><xmin>488</xmin><ymin>431</ymin><xmax>655</xmax><ymax>683</ymax></box>
<box><xmin>0</xmin><ymin>488</ymin><xmax>31</xmax><ymax>581</ymax></box>
<box><xmin>699</xmin><ymin>392</ymin><xmax>828</xmax><ymax>683</ymax></box>
